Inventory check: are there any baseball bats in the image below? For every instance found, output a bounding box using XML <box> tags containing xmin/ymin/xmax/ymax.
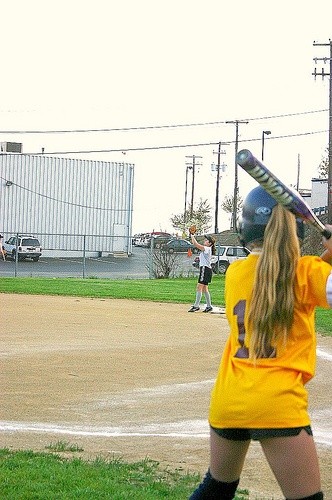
<box><xmin>236</xmin><ymin>149</ymin><xmax>332</xmax><ymax>240</ymax></box>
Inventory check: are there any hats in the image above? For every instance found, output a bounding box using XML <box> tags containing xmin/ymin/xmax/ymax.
<box><xmin>204</xmin><ymin>235</ymin><xmax>215</xmax><ymax>244</ymax></box>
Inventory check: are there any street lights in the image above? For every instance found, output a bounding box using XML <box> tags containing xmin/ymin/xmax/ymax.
<box><xmin>260</xmin><ymin>130</ymin><xmax>272</xmax><ymax>162</ymax></box>
<box><xmin>182</xmin><ymin>165</ymin><xmax>192</xmax><ymax>237</ymax></box>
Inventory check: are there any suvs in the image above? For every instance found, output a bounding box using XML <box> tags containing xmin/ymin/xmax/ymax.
<box><xmin>2</xmin><ymin>234</ymin><xmax>42</xmax><ymax>262</ymax></box>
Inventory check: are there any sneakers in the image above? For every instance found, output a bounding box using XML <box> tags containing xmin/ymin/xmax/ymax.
<box><xmin>188</xmin><ymin>305</ymin><xmax>200</xmax><ymax>312</ymax></box>
<box><xmin>202</xmin><ymin>306</ymin><xmax>213</xmax><ymax>312</ymax></box>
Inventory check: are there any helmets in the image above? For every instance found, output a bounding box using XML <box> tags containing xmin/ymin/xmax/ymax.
<box><xmin>239</xmin><ymin>186</ymin><xmax>304</xmax><ymax>245</ymax></box>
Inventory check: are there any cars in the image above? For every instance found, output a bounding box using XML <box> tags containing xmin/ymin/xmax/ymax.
<box><xmin>192</xmin><ymin>243</ymin><xmax>252</xmax><ymax>274</ymax></box>
<box><xmin>161</xmin><ymin>239</ymin><xmax>200</xmax><ymax>254</ymax></box>
<box><xmin>134</xmin><ymin>234</ymin><xmax>174</xmax><ymax>249</ymax></box>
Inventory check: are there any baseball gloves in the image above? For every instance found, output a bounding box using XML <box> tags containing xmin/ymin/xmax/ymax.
<box><xmin>189</xmin><ymin>225</ymin><xmax>196</xmax><ymax>235</ymax></box>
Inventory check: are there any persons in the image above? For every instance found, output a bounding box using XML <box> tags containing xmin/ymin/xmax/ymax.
<box><xmin>188</xmin><ymin>226</ymin><xmax>215</xmax><ymax>313</ymax></box>
<box><xmin>187</xmin><ymin>184</ymin><xmax>332</xmax><ymax>500</ymax></box>
